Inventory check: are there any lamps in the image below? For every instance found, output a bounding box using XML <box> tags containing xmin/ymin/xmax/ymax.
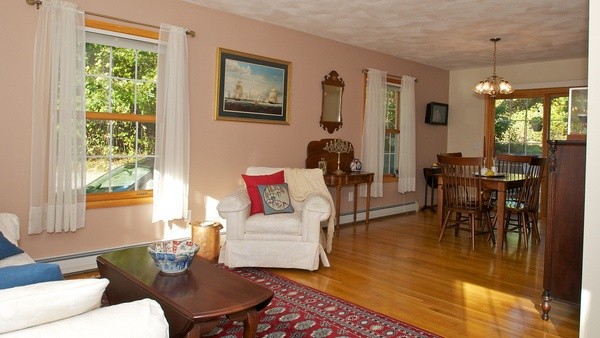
<box><xmin>473</xmin><ymin>36</ymin><xmax>515</xmax><ymax>96</ymax></box>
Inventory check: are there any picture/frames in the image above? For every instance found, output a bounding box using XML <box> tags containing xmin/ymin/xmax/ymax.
<box><xmin>214</xmin><ymin>47</ymin><xmax>293</xmax><ymax>125</ymax></box>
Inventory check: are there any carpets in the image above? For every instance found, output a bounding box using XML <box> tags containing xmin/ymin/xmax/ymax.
<box><xmin>203</xmin><ymin>258</ymin><xmax>446</xmax><ymax>338</ymax></box>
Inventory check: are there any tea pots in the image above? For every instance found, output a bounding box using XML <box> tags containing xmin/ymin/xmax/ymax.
<box><xmin>481</xmin><ymin>166</ymin><xmax>490</xmax><ymax>176</ymax></box>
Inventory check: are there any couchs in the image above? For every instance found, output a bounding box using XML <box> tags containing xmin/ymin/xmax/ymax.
<box><xmin>216</xmin><ymin>168</ymin><xmax>336</xmax><ymax>272</ymax></box>
<box><xmin>1</xmin><ymin>213</ymin><xmax>170</xmax><ymax>337</ymax></box>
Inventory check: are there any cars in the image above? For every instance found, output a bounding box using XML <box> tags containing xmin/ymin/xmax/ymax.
<box><xmin>86</xmin><ymin>157</ymin><xmax>154</xmax><ymax>193</ymax></box>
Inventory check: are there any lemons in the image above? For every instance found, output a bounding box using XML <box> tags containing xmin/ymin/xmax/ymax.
<box><xmin>487</xmin><ymin>171</ymin><xmax>494</xmax><ymax>176</ymax></box>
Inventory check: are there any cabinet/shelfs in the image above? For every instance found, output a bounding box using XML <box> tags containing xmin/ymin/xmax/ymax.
<box><xmin>541</xmin><ymin>138</ymin><xmax>586</xmax><ymax>324</ymax></box>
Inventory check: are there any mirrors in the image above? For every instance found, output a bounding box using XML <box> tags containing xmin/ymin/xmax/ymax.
<box><xmin>320</xmin><ymin>69</ymin><xmax>346</xmax><ymax>134</ymax></box>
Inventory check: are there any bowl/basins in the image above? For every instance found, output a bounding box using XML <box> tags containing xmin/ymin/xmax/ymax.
<box><xmin>147</xmin><ymin>238</ymin><xmax>200</xmax><ymax>275</ymax></box>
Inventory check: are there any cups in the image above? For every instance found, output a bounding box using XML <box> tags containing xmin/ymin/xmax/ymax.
<box><xmin>491</xmin><ymin>166</ymin><xmax>499</xmax><ymax>175</ymax></box>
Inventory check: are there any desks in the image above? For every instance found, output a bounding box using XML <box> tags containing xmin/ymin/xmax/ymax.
<box><xmin>438</xmin><ymin>173</ymin><xmax>540</xmax><ymax>252</ymax></box>
<box><xmin>305</xmin><ymin>138</ymin><xmax>375</xmax><ymax>232</ymax></box>
<box><xmin>422</xmin><ymin>166</ymin><xmax>444</xmax><ymax>213</ymax></box>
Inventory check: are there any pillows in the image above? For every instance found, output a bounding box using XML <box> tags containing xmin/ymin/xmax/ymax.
<box><xmin>0</xmin><ymin>263</ymin><xmax>64</xmax><ymax>290</ymax></box>
<box><xmin>256</xmin><ymin>183</ymin><xmax>293</xmax><ymax>214</ymax></box>
<box><xmin>241</xmin><ymin>170</ymin><xmax>284</xmax><ymax>215</ymax></box>
<box><xmin>0</xmin><ymin>231</ymin><xmax>25</xmax><ymax>259</ymax></box>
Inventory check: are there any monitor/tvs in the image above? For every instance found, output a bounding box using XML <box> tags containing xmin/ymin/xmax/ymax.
<box><xmin>425</xmin><ymin>102</ymin><xmax>448</xmax><ymax>125</ymax></box>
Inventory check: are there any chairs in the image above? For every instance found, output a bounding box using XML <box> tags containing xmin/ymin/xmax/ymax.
<box><xmin>487</xmin><ymin>153</ymin><xmax>539</xmax><ymax>242</ymax></box>
<box><xmin>437</xmin><ymin>152</ymin><xmax>489</xmax><ymax>236</ymax></box>
<box><xmin>437</xmin><ymin>155</ymin><xmax>496</xmax><ymax>250</ymax></box>
<box><xmin>486</xmin><ymin>156</ymin><xmax>547</xmax><ymax>248</ymax></box>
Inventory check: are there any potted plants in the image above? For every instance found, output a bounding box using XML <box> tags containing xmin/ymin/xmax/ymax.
<box><xmin>530</xmin><ymin>116</ymin><xmax>543</xmax><ymax>131</ymax></box>
